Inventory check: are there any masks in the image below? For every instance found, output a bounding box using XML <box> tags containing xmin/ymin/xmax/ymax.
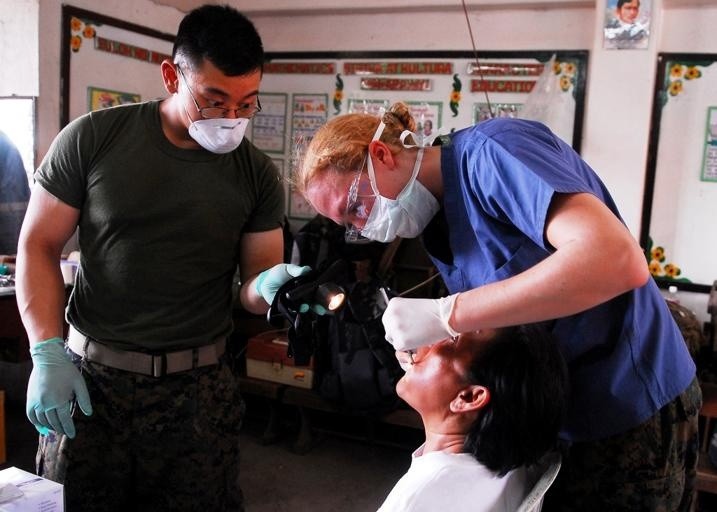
<box><xmin>178</xmin><ymin>77</ymin><xmax>251</xmax><ymax>154</ymax></box>
<box><xmin>362</xmin><ymin>152</ymin><xmax>441</xmax><ymax>244</ymax></box>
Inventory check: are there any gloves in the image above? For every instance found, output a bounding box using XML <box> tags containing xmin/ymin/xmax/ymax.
<box><xmin>382</xmin><ymin>293</ymin><xmax>462</xmax><ymax>352</ymax></box>
<box><xmin>26</xmin><ymin>336</ymin><xmax>93</xmax><ymax>439</ymax></box>
<box><xmin>255</xmin><ymin>263</ymin><xmax>327</xmax><ymax>316</ymax></box>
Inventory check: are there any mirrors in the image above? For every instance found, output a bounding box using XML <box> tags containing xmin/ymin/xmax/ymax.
<box><xmin>643</xmin><ymin>51</ymin><xmax>715</xmax><ymax>292</ymax></box>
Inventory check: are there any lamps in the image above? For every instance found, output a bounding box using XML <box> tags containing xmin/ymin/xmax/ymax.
<box><xmin>277</xmin><ymin>257</ymin><xmax>352</xmax><ymax>325</ymax></box>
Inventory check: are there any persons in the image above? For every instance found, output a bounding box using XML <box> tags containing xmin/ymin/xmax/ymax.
<box><xmin>0</xmin><ymin>130</ymin><xmax>30</xmax><ymax>259</ymax></box>
<box><xmin>14</xmin><ymin>2</ymin><xmax>329</xmax><ymax>512</ymax></box>
<box><xmin>295</xmin><ymin>101</ymin><xmax>704</xmax><ymax>512</ymax></box>
<box><xmin>381</xmin><ymin>328</ymin><xmax>581</xmax><ymax>512</ymax></box>
<box><xmin>604</xmin><ymin>0</ymin><xmax>648</xmax><ymax>42</ymax></box>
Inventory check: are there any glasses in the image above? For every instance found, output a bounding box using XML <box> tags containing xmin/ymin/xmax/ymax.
<box><xmin>178</xmin><ymin>64</ymin><xmax>261</xmax><ymax>119</ymax></box>
<box><xmin>344</xmin><ymin>158</ymin><xmax>365</xmax><ymax>244</ymax></box>
